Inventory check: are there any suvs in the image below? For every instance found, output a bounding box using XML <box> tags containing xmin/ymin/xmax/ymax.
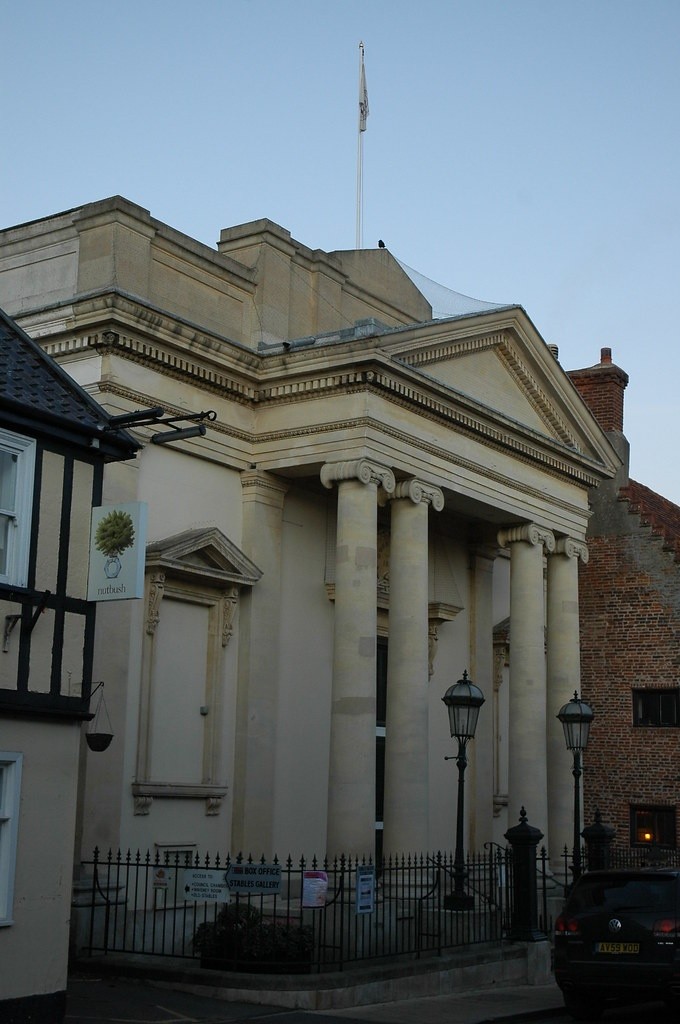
<box><xmin>551</xmin><ymin>868</ymin><xmax>680</xmax><ymax>1024</ymax></box>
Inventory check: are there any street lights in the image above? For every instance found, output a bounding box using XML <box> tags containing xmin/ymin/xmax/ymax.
<box><xmin>441</xmin><ymin>670</ymin><xmax>486</xmax><ymax>911</ymax></box>
<box><xmin>557</xmin><ymin>689</ymin><xmax>594</xmax><ymax>881</ymax></box>
